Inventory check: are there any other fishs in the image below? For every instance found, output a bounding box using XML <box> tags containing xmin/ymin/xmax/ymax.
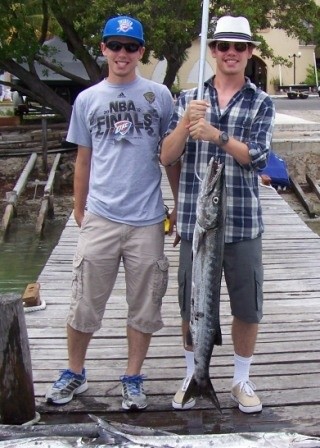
<box><xmin>177</xmin><ymin>154</ymin><xmax>227</xmax><ymax>416</ymax></box>
<box><xmin>0</xmin><ymin>412</ymin><xmax>320</xmax><ymax>448</ymax></box>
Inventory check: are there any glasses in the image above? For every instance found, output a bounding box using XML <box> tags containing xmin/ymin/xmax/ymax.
<box><xmin>215</xmin><ymin>42</ymin><xmax>250</xmax><ymax>52</ymax></box>
<box><xmin>105</xmin><ymin>41</ymin><xmax>142</xmax><ymax>52</ymax></box>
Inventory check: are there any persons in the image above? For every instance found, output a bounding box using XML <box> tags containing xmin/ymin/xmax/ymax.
<box><xmin>159</xmin><ymin>16</ymin><xmax>275</xmax><ymax>412</ymax></box>
<box><xmin>46</xmin><ymin>17</ymin><xmax>181</xmax><ymax>411</ymax></box>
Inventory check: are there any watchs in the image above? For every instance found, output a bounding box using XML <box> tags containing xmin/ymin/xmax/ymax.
<box><xmin>219</xmin><ymin>131</ymin><xmax>229</xmax><ymax>146</ymax></box>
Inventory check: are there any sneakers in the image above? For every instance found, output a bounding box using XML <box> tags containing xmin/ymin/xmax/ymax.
<box><xmin>46</xmin><ymin>366</ymin><xmax>88</xmax><ymax>403</ymax></box>
<box><xmin>171</xmin><ymin>378</ymin><xmax>198</xmax><ymax>410</ymax></box>
<box><xmin>232</xmin><ymin>380</ymin><xmax>263</xmax><ymax>412</ymax></box>
<box><xmin>119</xmin><ymin>373</ymin><xmax>147</xmax><ymax>410</ymax></box>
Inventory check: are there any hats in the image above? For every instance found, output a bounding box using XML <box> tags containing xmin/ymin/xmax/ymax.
<box><xmin>206</xmin><ymin>16</ymin><xmax>261</xmax><ymax>47</ymax></box>
<box><xmin>103</xmin><ymin>16</ymin><xmax>145</xmax><ymax>46</ymax></box>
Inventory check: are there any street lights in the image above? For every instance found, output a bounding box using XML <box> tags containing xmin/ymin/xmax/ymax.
<box><xmin>288</xmin><ymin>52</ymin><xmax>301</xmax><ymax>85</ymax></box>
